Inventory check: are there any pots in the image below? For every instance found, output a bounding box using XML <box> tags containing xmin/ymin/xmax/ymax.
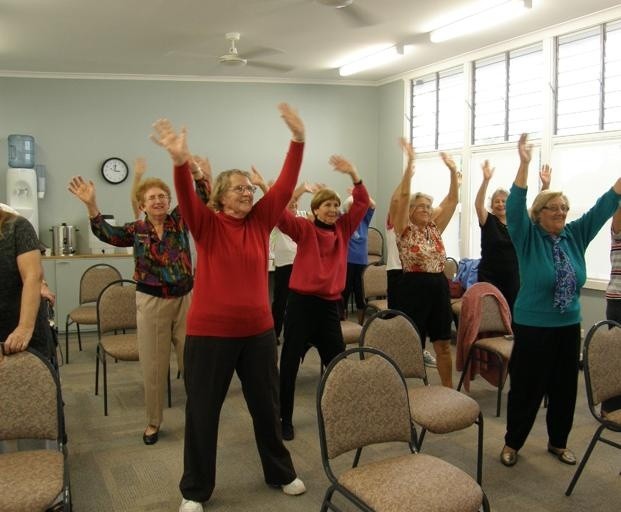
<box><xmin>49</xmin><ymin>221</ymin><xmax>79</xmax><ymax>256</ymax></box>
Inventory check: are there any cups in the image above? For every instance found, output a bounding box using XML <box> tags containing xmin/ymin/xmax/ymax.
<box><xmin>44</xmin><ymin>248</ymin><xmax>51</xmax><ymax>256</ymax></box>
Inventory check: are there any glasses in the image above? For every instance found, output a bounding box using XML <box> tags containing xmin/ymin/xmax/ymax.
<box><xmin>543</xmin><ymin>205</ymin><xmax>570</xmax><ymax>212</ymax></box>
<box><xmin>227</xmin><ymin>184</ymin><xmax>257</xmax><ymax>193</ymax></box>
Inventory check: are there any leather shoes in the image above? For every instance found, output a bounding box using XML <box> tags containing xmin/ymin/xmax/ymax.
<box><xmin>142</xmin><ymin>424</ymin><xmax>159</xmax><ymax>445</ymax></box>
<box><xmin>500</xmin><ymin>445</ymin><xmax>517</xmax><ymax>467</ymax></box>
<box><xmin>547</xmin><ymin>445</ymin><xmax>577</xmax><ymax>465</ymax></box>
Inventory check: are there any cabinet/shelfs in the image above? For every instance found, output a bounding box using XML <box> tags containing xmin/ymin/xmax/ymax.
<box><xmin>43</xmin><ymin>252</ymin><xmax>133</xmax><ymax>334</ymax></box>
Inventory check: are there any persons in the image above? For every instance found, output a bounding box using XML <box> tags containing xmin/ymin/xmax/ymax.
<box><xmin>597</xmin><ymin>202</ymin><xmax>621</xmax><ymax>430</ymax></box>
<box><xmin>68</xmin><ymin>157</ymin><xmax>210</xmax><ymax>447</ymax></box>
<box><xmin>0</xmin><ymin>209</ymin><xmax>68</xmax><ymax>456</ymax></box>
<box><xmin>498</xmin><ymin>132</ymin><xmax>621</xmax><ymax>467</ymax></box>
<box><xmin>385</xmin><ymin>166</ymin><xmax>464</xmax><ymax>369</ymax></box>
<box><xmin>387</xmin><ymin>136</ymin><xmax>454</xmax><ymax>391</ymax></box>
<box><xmin>149</xmin><ymin>102</ymin><xmax>307</xmax><ymax>512</ymax></box>
<box><xmin>0</xmin><ymin>203</ymin><xmax>55</xmax><ymax>308</ymax></box>
<box><xmin>130</xmin><ymin>159</ymin><xmax>211</xmax><ymax>217</ymax></box>
<box><xmin>247</xmin><ymin>154</ymin><xmax>370</xmax><ymax>442</ymax></box>
<box><xmin>271</xmin><ymin>176</ymin><xmax>329</xmax><ymax>346</ymax></box>
<box><xmin>341</xmin><ymin>186</ymin><xmax>375</xmax><ymax>324</ymax></box>
<box><xmin>473</xmin><ymin>159</ymin><xmax>552</xmax><ymax>327</ymax></box>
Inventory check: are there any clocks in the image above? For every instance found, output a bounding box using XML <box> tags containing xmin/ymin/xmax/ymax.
<box><xmin>99</xmin><ymin>154</ymin><xmax>130</xmax><ymax>186</ymax></box>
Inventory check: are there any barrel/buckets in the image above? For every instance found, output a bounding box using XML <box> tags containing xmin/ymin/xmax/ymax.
<box><xmin>7</xmin><ymin>134</ymin><xmax>35</xmax><ymax>169</ymax></box>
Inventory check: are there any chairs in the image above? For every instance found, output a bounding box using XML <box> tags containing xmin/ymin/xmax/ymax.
<box><xmin>351</xmin><ymin>309</ymin><xmax>485</xmax><ymax>485</ymax></box>
<box><xmin>441</xmin><ymin>256</ymin><xmax>459</xmax><ymax>288</ymax></box>
<box><xmin>367</xmin><ymin>227</ymin><xmax>386</xmax><ymax>272</ymax></box>
<box><xmin>64</xmin><ymin>262</ymin><xmax>124</xmax><ymax>364</ymax></box>
<box><xmin>358</xmin><ymin>261</ymin><xmax>391</xmax><ymax>326</ymax></box>
<box><xmin>318</xmin><ymin>318</ymin><xmax>365</xmax><ymax>383</ymax></box>
<box><xmin>310</xmin><ymin>347</ymin><xmax>490</xmax><ymax>512</ymax></box>
<box><xmin>454</xmin><ymin>279</ymin><xmax>517</xmax><ymax>418</ymax></box>
<box><xmin>563</xmin><ymin>319</ymin><xmax>621</xmax><ymax>498</ymax></box>
<box><xmin>93</xmin><ymin>279</ymin><xmax>174</xmax><ymax>417</ymax></box>
<box><xmin>1</xmin><ymin>334</ymin><xmax>74</xmax><ymax>512</ymax></box>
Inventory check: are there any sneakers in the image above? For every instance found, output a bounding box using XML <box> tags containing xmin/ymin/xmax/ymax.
<box><xmin>423</xmin><ymin>350</ymin><xmax>438</xmax><ymax>368</ymax></box>
<box><xmin>281</xmin><ymin>421</ymin><xmax>294</xmax><ymax>442</ymax></box>
<box><xmin>281</xmin><ymin>477</ymin><xmax>306</xmax><ymax>495</ymax></box>
<box><xmin>178</xmin><ymin>498</ymin><xmax>203</xmax><ymax>512</ymax></box>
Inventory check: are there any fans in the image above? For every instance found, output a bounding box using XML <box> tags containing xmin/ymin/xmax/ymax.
<box><xmin>163</xmin><ymin>28</ymin><xmax>300</xmax><ymax>80</ymax></box>
<box><xmin>295</xmin><ymin>1</ymin><xmax>382</xmax><ymax>33</ymax></box>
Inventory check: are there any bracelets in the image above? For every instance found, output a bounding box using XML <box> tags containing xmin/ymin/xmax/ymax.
<box><xmin>352</xmin><ymin>178</ymin><xmax>362</xmax><ymax>186</ymax></box>
<box><xmin>40</xmin><ymin>278</ymin><xmax>48</xmax><ymax>287</ymax></box>
<box><xmin>189</xmin><ymin>166</ymin><xmax>201</xmax><ymax>175</ymax></box>
